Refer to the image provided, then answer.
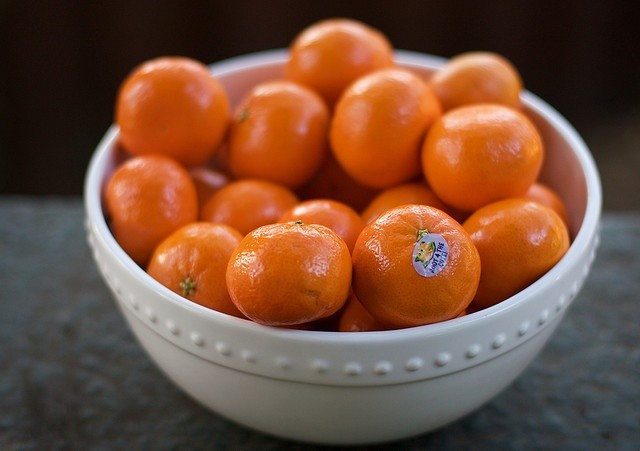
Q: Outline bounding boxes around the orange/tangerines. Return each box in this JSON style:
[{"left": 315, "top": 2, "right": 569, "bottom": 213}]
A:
[{"left": 103, "top": 16, "right": 571, "bottom": 332}]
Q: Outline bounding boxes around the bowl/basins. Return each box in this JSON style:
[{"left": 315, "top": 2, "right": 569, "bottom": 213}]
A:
[{"left": 82, "top": 52, "right": 602, "bottom": 443}]
